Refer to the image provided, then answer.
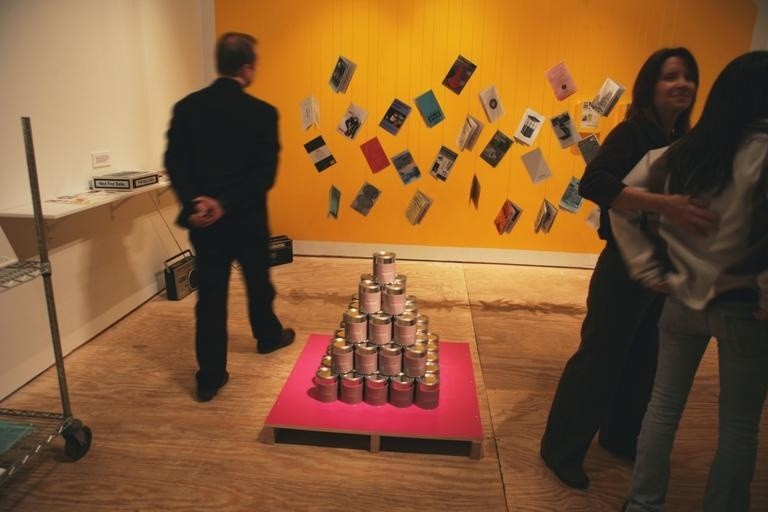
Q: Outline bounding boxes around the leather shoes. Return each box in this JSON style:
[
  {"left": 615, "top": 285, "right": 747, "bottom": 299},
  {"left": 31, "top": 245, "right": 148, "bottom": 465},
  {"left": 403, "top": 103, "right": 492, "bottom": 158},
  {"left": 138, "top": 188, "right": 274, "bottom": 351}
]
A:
[
  {"left": 541, "top": 433, "right": 636, "bottom": 488},
  {"left": 258, "top": 329, "right": 294, "bottom": 353},
  {"left": 197, "top": 371, "right": 228, "bottom": 401}
]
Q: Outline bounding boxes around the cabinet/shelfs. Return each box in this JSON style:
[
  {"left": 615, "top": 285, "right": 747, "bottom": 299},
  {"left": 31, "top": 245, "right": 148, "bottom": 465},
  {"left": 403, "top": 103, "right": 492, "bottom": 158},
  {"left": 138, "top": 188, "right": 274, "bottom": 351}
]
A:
[{"left": 0, "top": 116, "right": 94, "bottom": 490}]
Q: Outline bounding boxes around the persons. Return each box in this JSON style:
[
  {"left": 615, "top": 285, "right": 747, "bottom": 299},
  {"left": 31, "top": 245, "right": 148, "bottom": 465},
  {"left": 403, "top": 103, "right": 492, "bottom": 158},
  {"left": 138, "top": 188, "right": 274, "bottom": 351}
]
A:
[
  {"left": 609, "top": 50, "right": 768, "bottom": 512},
  {"left": 163, "top": 33, "right": 295, "bottom": 402},
  {"left": 540, "top": 47, "right": 720, "bottom": 488}
]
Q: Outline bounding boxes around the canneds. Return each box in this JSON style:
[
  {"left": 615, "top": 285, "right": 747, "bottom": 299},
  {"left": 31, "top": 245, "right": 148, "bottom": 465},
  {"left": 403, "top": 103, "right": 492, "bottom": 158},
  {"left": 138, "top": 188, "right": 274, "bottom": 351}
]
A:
[{"left": 316, "top": 251, "right": 438, "bottom": 409}]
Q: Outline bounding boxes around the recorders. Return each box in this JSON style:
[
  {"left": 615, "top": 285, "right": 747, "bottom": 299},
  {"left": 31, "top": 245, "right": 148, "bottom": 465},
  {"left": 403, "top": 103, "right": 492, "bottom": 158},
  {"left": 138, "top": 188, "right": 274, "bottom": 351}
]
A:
[
  {"left": 163, "top": 249, "right": 201, "bottom": 301},
  {"left": 268, "top": 234, "right": 293, "bottom": 267}
]
